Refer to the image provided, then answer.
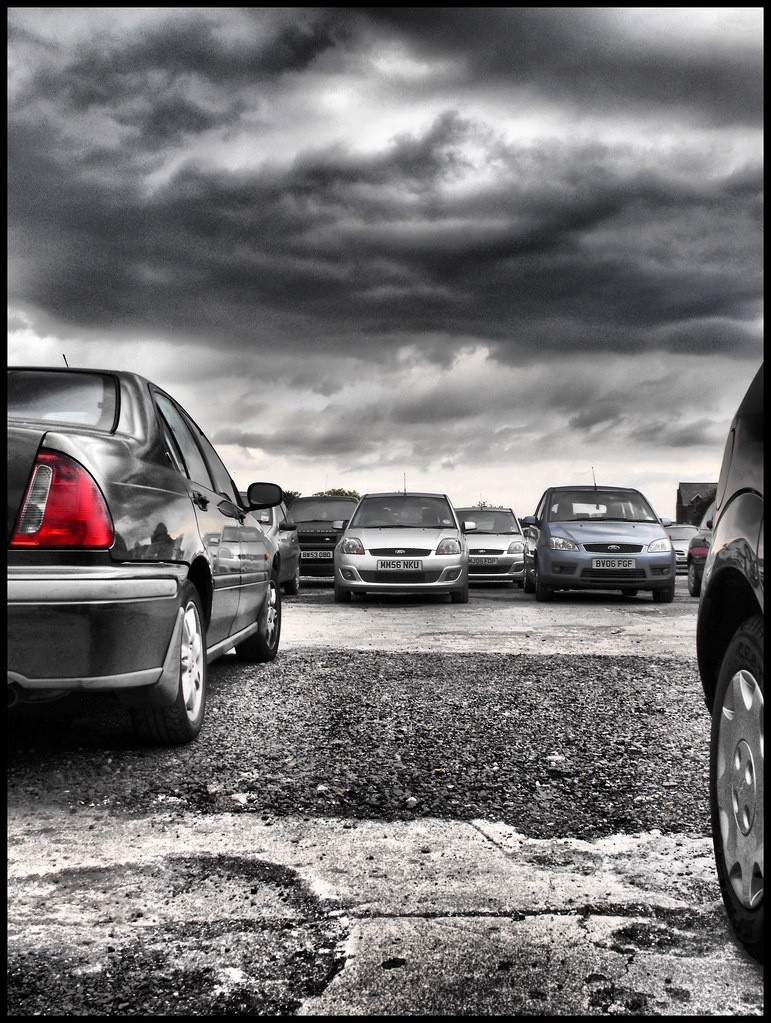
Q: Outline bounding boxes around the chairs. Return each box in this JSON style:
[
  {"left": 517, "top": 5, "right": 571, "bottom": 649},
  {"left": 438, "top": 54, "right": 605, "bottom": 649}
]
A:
[
  {"left": 607, "top": 504, "right": 629, "bottom": 519},
  {"left": 365, "top": 510, "right": 387, "bottom": 521},
  {"left": 557, "top": 500, "right": 576, "bottom": 520},
  {"left": 492, "top": 516, "right": 510, "bottom": 531},
  {"left": 423, "top": 507, "right": 439, "bottom": 522}
]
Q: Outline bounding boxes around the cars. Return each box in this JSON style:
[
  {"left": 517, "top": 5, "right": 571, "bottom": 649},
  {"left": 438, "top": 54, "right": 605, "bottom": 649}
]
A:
[
  {"left": 452, "top": 505, "right": 529, "bottom": 590},
  {"left": 331, "top": 491, "right": 478, "bottom": 604},
  {"left": 663, "top": 523, "right": 699, "bottom": 569},
  {"left": 695, "top": 357, "right": 766, "bottom": 964},
  {"left": 687, "top": 500, "right": 717, "bottom": 597},
  {"left": 279, "top": 494, "right": 362, "bottom": 576},
  {"left": 523, "top": 485, "right": 677, "bottom": 603},
  {"left": 237, "top": 490, "right": 301, "bottom": 595},
  {"left": 6, "top": 365, "right": 283, "bottom": 750}
]
